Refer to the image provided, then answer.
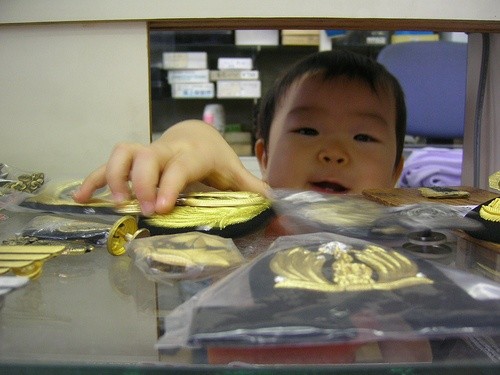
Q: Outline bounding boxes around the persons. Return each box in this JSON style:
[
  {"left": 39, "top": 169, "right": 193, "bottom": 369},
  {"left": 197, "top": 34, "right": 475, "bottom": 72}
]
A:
[{"left": 72, "top": 50, "right": 407, "bottom": 220}]
